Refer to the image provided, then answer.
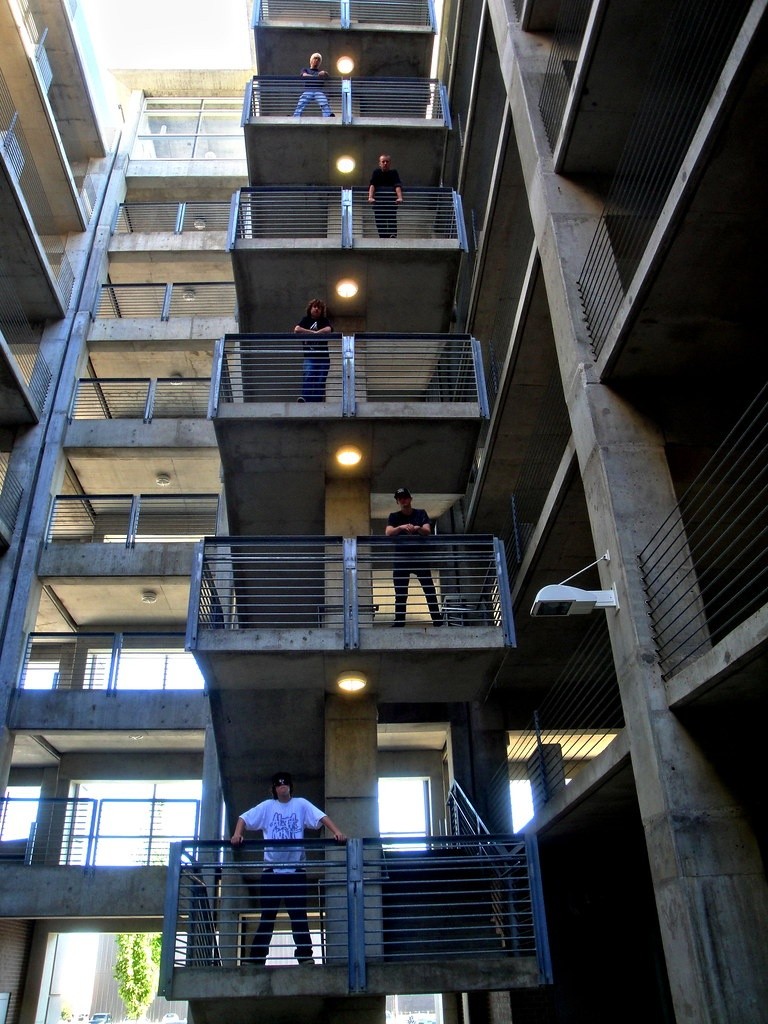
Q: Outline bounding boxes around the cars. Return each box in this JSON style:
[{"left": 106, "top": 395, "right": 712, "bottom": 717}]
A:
[
  {"left": 162, "top": 1013, "right": 181, "bottom": 1024},
  {"left": 88, "top": 1011, "right": 115, "bottom": 1024}
]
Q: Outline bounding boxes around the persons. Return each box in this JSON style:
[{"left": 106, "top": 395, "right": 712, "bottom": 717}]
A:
[
  {"left": 293, "top": 53, "right": 336, "bottom": 117},
  {"left": 368, "top": 151, "right": 402, "bottom": 238},
  {"left": 231, "top": 772, "right": 347, "bottom": 965},
  {"left": 294, "top": 298, "right": 333, "bottom": 402},
  {"left": 385, "top": 487, "right": 444, "bottom": 627}
]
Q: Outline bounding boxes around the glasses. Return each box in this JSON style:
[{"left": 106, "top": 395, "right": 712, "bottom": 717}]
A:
[{"left": 275, "top": 780, "right": 291, "bottom": 786}]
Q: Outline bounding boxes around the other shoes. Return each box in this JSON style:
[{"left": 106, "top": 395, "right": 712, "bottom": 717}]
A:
[
  {"left": 298, "top": 956, "right": 314, "bottom": 965},
  {"left": 241, "top": 961, "right": 264, "bottom": 966},
  {"left": 391, "top": 624, "right": 404, "bottom": 627},
  {"left": 297, "top": 398, "right": 305, "bottom": 402},
  {"left": 389, "top": 234, "right": 396, "bottom": 238}
]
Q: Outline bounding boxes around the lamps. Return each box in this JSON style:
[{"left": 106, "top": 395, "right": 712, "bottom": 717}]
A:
[
  {"left": 194, "top": 219, "right": 207, "bottom": 230},
  {"left": 169, "top": 375, "right": 183, "bottom": 386},
  {"left": 204, "top": 151, "right": 216, "bottom": 159},
  {"left": 182, "top": 290, "right": 194, "bottom": 302},
  {"left": 141, "top": 592, "right": 157, "bottom": 604},
  {"left": 530, "top": 550, "right": 621, "bottom": 618},
  {"left": 155, "top": 474, "right": 171, "bottom": 486},
  {"left": 336, "top": 671, "right": 368, "bottom": 692}
]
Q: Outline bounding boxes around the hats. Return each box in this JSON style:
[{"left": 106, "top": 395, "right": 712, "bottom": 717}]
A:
[{"left": 394, "top": 487, "right": 412, "bottom": 498}]
